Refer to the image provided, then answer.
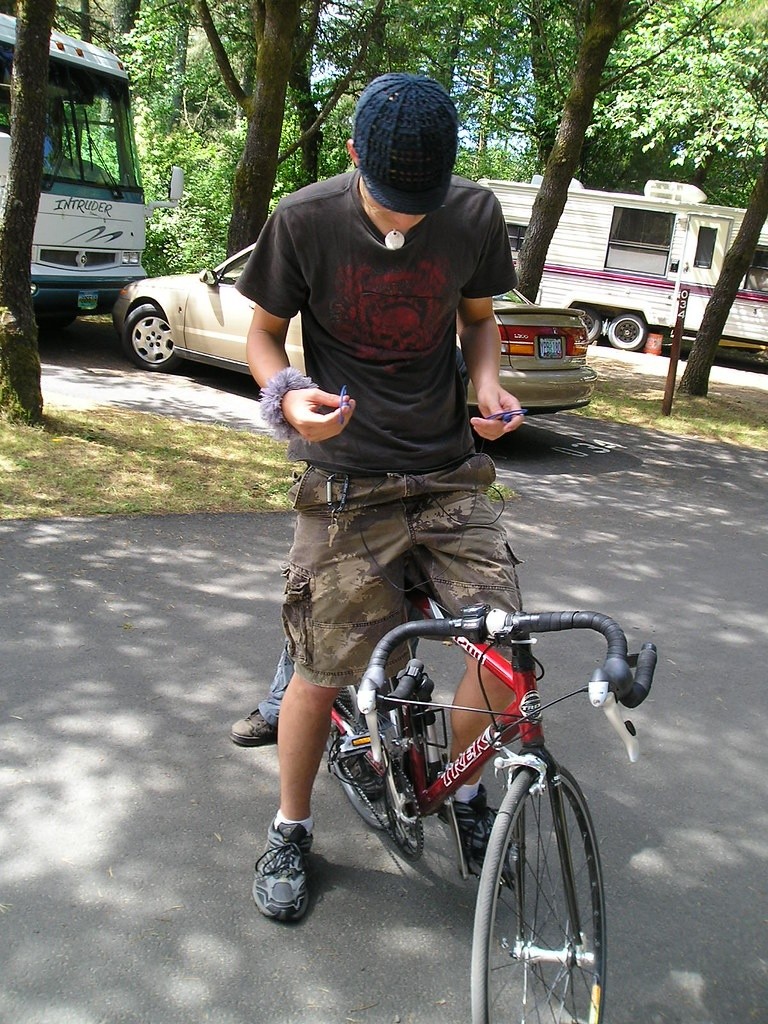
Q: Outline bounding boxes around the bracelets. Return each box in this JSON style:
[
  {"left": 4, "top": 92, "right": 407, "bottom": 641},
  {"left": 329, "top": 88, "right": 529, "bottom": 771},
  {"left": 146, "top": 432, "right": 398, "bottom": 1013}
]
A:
[{"left": 258, "top": 364, "right": 319, "bottom": 441}]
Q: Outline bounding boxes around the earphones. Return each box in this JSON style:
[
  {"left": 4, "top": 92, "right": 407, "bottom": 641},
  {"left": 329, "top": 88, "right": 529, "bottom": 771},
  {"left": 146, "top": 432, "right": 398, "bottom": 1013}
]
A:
[
  {"left": 341, "top": 388, "right": 350, "bottom": 406},
  {"left": 502, "top": 413, "right": 513, "bottom": 423}
]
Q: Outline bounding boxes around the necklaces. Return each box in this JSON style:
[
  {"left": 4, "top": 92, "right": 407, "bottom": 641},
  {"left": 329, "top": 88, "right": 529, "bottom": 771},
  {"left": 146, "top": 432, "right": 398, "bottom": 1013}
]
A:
[{"left": 364, "top": 195, "right": 404, "bottom": 250}]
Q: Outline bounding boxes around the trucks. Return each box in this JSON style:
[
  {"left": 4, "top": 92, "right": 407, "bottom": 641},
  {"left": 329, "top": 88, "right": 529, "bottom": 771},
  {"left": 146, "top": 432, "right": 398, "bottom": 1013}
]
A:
[
  {"left": 0, "top": 13, "right": 180, "bottom": 346},
  {"left": 474, "top": 173, "right": 768, "bottom": 370}
]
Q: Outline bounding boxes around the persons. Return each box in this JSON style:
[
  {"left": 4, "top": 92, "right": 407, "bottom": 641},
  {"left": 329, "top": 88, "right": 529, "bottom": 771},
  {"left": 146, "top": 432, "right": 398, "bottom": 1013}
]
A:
[{"left": 229, "top": 72, "right": 524, "bottom": 924}]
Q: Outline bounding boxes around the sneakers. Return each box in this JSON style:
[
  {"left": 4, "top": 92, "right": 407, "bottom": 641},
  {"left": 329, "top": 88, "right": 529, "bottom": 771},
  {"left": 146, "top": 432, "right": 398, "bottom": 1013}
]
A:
[
  {"left": 253, "top": 814, "right": 313, "bottom": 921},
  {"left": 229, "top": 708, "right": 279, "bottom": 747},
  {"left": 439, "top": 784, "right": 519, "bottom": 874}
]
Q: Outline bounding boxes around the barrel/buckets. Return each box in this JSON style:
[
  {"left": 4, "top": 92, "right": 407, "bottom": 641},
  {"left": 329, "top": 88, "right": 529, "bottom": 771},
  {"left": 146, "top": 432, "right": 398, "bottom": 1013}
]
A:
[{"left": 643, "top": 333, "right": 664, "bottom": 354}]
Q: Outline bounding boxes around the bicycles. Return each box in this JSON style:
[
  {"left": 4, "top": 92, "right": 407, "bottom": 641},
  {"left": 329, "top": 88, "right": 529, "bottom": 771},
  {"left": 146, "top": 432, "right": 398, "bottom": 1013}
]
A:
[{"left": 327, "top": 544, "right": 661, "bottom": 1024}]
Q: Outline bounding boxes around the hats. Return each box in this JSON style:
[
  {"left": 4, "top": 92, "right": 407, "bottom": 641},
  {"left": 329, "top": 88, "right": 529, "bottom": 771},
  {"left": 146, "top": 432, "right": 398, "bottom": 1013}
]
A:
[{"left": 353, "top": 72, "right": 457, "bottom": 214}]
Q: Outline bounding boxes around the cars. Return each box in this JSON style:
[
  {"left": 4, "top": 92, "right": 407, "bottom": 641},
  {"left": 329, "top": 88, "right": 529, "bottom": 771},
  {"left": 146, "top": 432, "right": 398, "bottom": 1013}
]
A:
[{"left": 114, "top": 240, "right": 597, "bottom": 443}]
